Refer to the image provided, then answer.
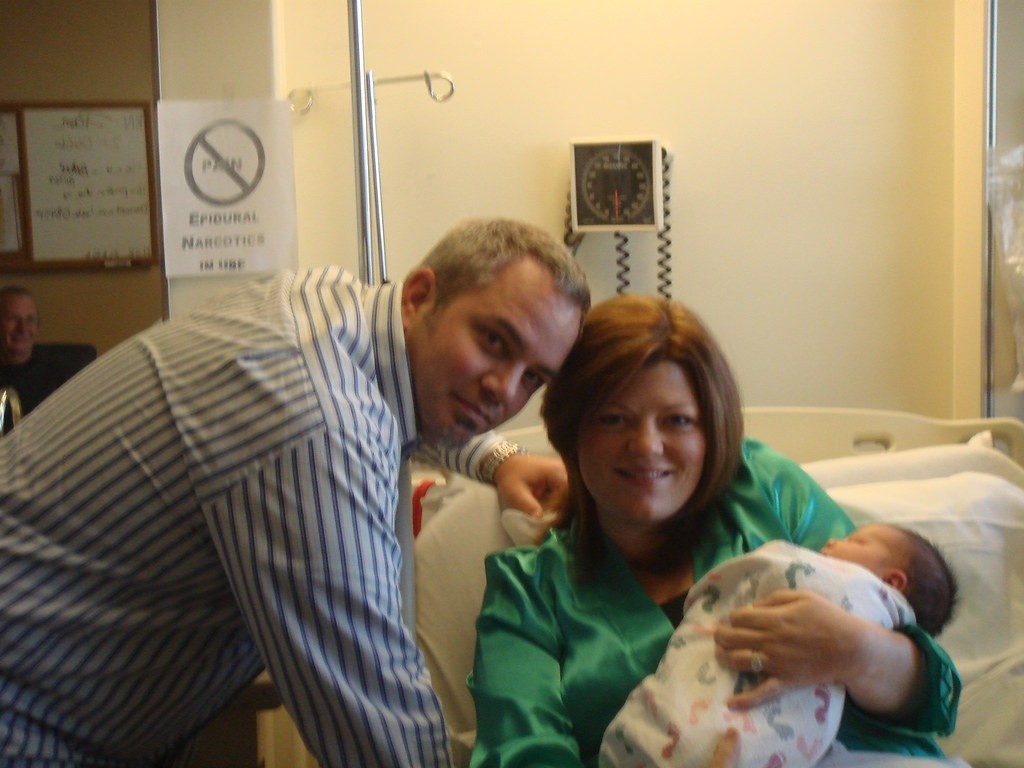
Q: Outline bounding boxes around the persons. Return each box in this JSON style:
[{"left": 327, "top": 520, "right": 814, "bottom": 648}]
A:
[
  {"left": 465, "top": 290, "right": 963, "bottom": 768},
  {"left": 0, "top": 220, "right": 591, "bottom": 768},
  {"left": 0, "top": 284, "right": 43, "bottom": 437}
]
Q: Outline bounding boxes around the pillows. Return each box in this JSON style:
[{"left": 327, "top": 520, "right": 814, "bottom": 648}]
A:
[{"left": 415, "top": 432, "right": 1024, "bottom": 768}]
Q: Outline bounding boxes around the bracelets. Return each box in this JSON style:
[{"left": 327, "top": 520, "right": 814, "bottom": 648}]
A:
[{"left": 483, "top": 441, "right": 521, "bottom": 484}]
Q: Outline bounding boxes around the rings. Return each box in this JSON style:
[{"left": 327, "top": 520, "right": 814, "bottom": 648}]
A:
[{"left": 751, "top": 648, "right": 763, "bottom": 673}]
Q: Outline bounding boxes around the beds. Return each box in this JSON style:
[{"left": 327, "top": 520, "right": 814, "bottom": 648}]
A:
[{"left": 410, "top": 406, "right": 1024, "bottom": 768}]
